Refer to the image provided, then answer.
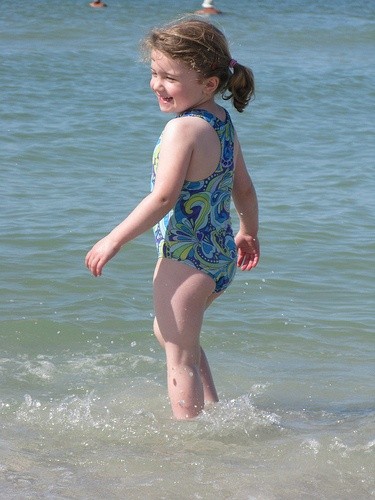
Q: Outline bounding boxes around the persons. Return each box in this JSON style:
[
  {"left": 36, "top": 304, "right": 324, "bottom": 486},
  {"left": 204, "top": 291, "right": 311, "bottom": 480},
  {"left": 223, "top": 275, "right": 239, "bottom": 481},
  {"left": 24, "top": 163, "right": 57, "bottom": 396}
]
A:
[
  {"left": 195, "top": 0, "right": 223, "bottom": 14},
  {"left": 89, "top": 0, "right": 107, "bottom": 8},
  {"left": 84, "top": 19, "right": 260, "bottom": 421}
]
